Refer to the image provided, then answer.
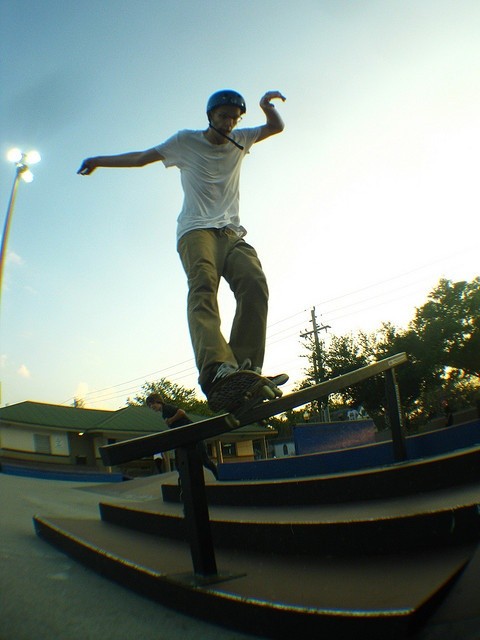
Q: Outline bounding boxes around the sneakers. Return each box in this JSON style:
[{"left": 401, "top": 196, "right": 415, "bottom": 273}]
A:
[
  {"left": 223, "top": 373, "right": 289, "bottom": 414},
  {"left": 200, "top": 359, "right": 261, "bottom": 394}
]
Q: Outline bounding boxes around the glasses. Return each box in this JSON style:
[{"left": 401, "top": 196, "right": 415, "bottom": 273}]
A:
[{"left": 214, "top": 111, "right": 242, "bottom": 123}]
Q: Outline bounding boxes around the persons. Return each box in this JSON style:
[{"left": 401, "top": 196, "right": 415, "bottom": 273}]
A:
[
  {"left": 144, "top": 392, "right": 219, "bottom": 483},
  {"left": 442, "top": 401, "right": 454, "bottom": 427},
  {"left": 75, "top": 89, "right": 290, "bottom": 408},
  {"left": 152, "top": 452, "right": 163, "bottom": 476}
]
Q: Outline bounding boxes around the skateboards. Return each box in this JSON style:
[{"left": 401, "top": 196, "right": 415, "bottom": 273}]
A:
[{"left": 207, "top": 370, "right": 283, "bottom": 429}]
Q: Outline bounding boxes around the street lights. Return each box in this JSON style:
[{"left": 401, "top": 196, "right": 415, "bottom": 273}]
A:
[{"left": 0, "top": 149, "right": 41, "bottom": 286}]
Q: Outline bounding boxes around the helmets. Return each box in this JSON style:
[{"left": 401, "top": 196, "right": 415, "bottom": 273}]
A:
[{"left": 207, "top": 89, "right": 246, "bottom": 115}]
